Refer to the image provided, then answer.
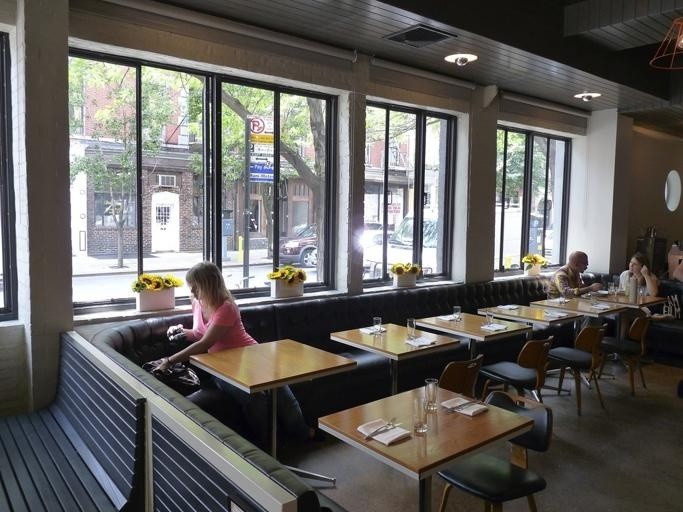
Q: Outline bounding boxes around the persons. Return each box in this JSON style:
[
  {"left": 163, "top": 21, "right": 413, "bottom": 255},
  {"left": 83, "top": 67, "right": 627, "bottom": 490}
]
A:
[
  {"left": 617, "top": 252, "right": 658, "bottom": 341},
  {"left": 153, "top": 264, "right": 325, "bottom": 447},
  {"left": 546, "top": 252, "right": 602, "bottom": 338}
]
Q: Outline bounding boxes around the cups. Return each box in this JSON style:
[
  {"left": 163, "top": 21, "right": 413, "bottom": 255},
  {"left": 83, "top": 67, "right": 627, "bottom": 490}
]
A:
[
  {"left": 485, "top": 308, "right": 493, "bottom": 329},
  {"left": 591, "top": 292, "right": 597, "bottom": 305},
  {"left": 453, "top": 306, "right": 461, "bottom": 324},
  {"left": 424, "top": 379, "right": 438, "bottom": 412},
  {"left": 559, "top": 291, "right": 565, "bottom": 305},
  {"left": 638, "top": 287, "right": 644, "bottom": 300},
  {"left": 412, "top": 397, "right": 429, "bottom": 433},
  {"left": 373, "top": 317, "right": 382, "bottom": 337},
  {"left": 608, "top": 282, "right": 614, "bottom": 295},
  {"left": 407, "top": 318, "right": 416, "bottom": 341}
]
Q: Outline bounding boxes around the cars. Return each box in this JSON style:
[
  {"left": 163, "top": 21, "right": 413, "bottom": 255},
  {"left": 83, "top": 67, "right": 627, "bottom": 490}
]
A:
[{"left": 268, "top": 222, "right": 383, "bottom": 267}]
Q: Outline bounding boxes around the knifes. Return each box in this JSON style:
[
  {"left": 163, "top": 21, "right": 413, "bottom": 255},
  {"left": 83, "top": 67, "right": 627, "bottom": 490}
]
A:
[{"left": 367, "top": 422, "right": 403, "bottom": 440}]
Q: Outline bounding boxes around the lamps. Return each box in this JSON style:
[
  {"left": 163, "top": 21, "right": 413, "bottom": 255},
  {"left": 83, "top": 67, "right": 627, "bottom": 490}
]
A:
[
  {"left": 648, "top": 14, "right": 683, "bottom": 74},
  {"left": 443, "top": 52, "right": 479, "bottom": 68},
  {"left": 572, "top": 92, "right": 602, "bottom": 103}
]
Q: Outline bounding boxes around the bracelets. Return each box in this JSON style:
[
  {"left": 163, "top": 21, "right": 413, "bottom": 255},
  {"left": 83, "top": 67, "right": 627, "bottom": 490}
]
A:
[{"left": 166, "top": 358, "right": 173, "bottom": 366}]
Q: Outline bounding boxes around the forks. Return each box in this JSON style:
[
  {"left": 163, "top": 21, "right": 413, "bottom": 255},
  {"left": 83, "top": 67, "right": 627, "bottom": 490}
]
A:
[
  {"left": 447, "top": 400, "right": 487, "bottom": 414},
  {"left": 364, "top": 418, "right": 400, "bottom": 444}
]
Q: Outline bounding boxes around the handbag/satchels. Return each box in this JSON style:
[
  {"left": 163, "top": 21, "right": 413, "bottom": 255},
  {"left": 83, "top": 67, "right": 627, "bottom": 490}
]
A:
[{"left": 146, "top": 360, "right": 201, "bottom": 395}]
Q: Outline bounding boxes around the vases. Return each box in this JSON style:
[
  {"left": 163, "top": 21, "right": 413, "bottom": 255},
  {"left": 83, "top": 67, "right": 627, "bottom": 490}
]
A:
[
  {"left": 523, "top": 263, "right": 542, "bottom": 276},
  {"left": 135, "top": 287, "right": 177, "bottom": 314},
  {"left": 270, "top": 279, "right": 304, "bottom": 299},
  {"left": 392, "top": 274, "right": 417, "bottom": 288}
]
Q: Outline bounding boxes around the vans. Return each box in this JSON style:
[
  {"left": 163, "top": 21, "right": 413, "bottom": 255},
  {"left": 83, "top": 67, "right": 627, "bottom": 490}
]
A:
[{"left": 365, "top": 206, "right": 521, "bottom": 280}]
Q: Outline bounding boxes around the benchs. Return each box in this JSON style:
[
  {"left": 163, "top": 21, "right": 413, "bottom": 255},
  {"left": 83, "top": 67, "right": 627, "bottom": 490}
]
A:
[{"left": 0, "top": 330, "right": 300, "bottom": 512}]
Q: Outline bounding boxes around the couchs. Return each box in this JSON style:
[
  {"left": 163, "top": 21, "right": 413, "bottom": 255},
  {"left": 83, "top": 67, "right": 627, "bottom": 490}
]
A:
[{"left": 75, "top": 268, "right": 682, "bottom": 512}]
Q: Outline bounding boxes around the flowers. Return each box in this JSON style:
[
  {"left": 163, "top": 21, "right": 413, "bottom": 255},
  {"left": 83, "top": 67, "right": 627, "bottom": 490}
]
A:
[
  {"left": 132, "top": 273, "right": 183, "bottom": 293},
  {"left": 390, "top": 262, "right": 422, "bottom": 276},
  {"left": 266, "top": 265, "right": 307, "bottom": 286},
  {"left": 521, "top": 253, "right": 551, "bottom": 269}
]
